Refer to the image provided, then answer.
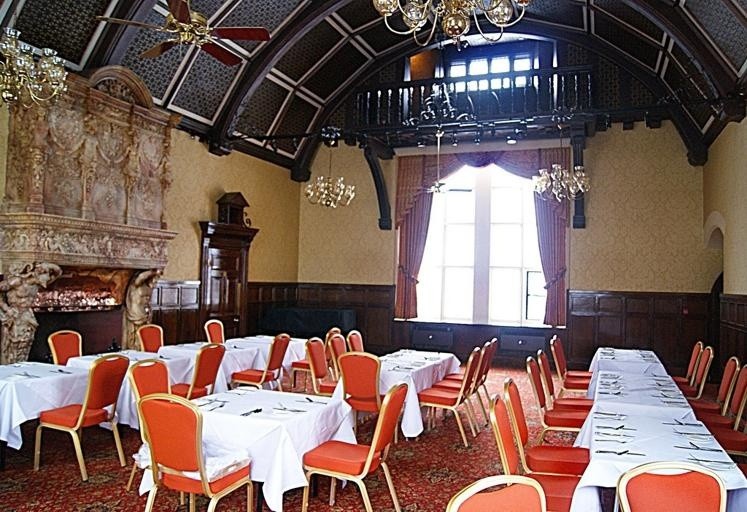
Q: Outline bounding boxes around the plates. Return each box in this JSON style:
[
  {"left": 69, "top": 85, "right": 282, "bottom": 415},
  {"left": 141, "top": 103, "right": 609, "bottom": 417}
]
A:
[
  {"left": 377, "top": 349, "right": 441, "bottom": 372},
  {"left": 95, "top": 349, "right": 160, "bottom": 364}
]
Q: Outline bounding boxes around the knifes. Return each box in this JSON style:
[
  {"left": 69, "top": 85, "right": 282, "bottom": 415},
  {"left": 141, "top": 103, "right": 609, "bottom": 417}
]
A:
[
  {"left": 662, "top": 419, "right": 738, "bottom": 470},
  {"left": 14, "top": 362, "right": 72, "bottom": 378},
  {"left": 594, "top": 411, "right": 646, "bottom": 472}
]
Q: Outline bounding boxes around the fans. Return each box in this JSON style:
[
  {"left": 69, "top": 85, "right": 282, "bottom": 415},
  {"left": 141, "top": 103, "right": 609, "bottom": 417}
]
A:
[
  {"left": 396, "top": 129, "right": 474, "bottom": 199},
  {"left": 89, "top": 0, "right": 271, "bottom": 68}
]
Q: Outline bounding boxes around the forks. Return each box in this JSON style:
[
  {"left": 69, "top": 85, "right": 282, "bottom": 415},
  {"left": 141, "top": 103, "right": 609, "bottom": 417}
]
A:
[
  {"left": 234, "top": 346, "right": 243, "bottom": 349},
  {"left": 158, "top": 355, "right": 171, "bottom": 360},
  {"left": 199, "top": 387, "right": 327, "bottom": 417},
  {"left": 598, "top": 347, "right": 688, "bottom": 408}
]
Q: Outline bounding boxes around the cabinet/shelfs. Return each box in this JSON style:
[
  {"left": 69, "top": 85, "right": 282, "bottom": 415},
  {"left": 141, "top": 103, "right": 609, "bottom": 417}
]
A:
[{"left": 391, "top": 317, "right": 568, "bottom": 370}]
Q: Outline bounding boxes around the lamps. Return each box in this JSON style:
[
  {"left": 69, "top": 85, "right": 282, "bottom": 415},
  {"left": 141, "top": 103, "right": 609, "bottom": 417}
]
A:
[
  {"left": 524, "top": 105, "right": 591, "bottom": 204},
  {"left": 372, "top": 0, "right": 532, "bottom": 52},
  {"left": 0, "top": 9, "right": 70, "bottom": 113},
  {"left": 303, "top": 126, "right": 357, "bottom": 209}
]
